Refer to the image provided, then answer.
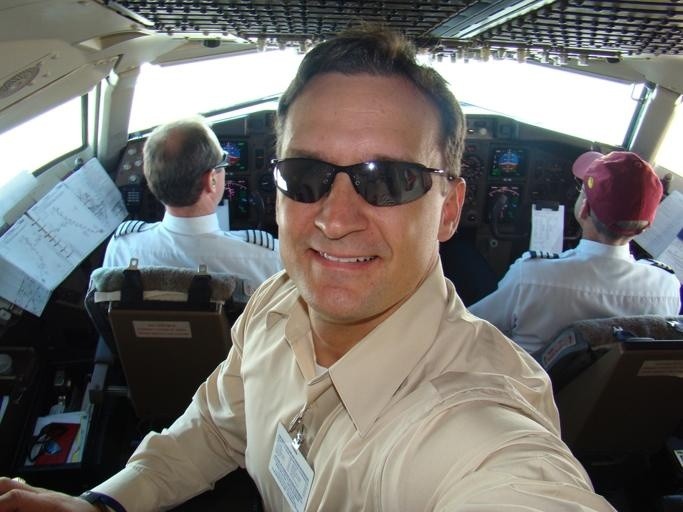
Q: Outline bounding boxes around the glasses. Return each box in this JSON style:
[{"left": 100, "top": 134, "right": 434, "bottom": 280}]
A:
[
  {"left": 269, "top": 155, "right": 455, "bottom": 208},
  {"left": 202, "top": 149, "right": 231, "bottom": 177}
]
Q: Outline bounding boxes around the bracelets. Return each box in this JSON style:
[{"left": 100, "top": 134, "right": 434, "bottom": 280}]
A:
[{"left": 79, "top": 490, "right": 126, "bottom": 512}]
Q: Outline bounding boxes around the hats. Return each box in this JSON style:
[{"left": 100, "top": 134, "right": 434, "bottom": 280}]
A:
[{"left": 573, "top": 149, "right": 665, "bottom": 236}]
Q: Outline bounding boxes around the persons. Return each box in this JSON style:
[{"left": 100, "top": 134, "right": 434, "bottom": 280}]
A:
[
  {"left": 465, "top": 148, "right": 682, "bottom": 356},
  {"left": 1, "top": 25, "right": 623, "bottom": 512},
  {"left": 100, "top": 112, "right": 282, "bottom": 304}
]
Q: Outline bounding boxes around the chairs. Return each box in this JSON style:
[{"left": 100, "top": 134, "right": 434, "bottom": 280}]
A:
[
  {"left": 531, "top": 316, "right": 683, "bottom": 458},
  {"left": 84, "top": 264, "right": 256, "bottom": 421}
]
